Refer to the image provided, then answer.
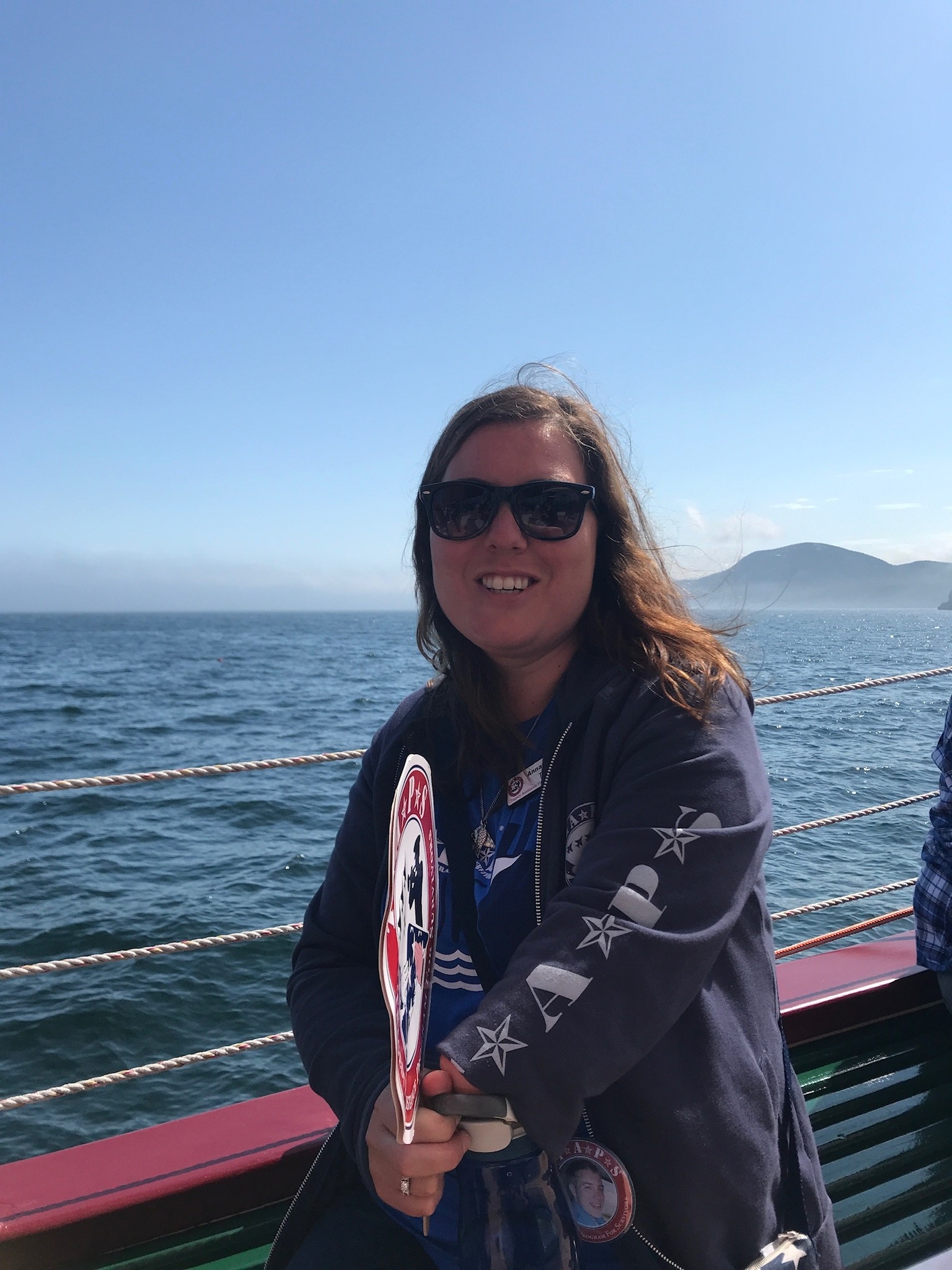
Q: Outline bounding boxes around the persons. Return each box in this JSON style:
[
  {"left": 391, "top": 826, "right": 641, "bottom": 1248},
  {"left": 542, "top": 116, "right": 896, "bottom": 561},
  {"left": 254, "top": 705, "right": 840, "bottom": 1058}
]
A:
[
  {"left": 912, "top": 695, "right": 952, "bottom": 1016},
  {"left": 565, "top": 1159, "right": 607, "bottom": 1226},
  {"left": 260, "top": 382, "right": 843, "bottom": 1270}
]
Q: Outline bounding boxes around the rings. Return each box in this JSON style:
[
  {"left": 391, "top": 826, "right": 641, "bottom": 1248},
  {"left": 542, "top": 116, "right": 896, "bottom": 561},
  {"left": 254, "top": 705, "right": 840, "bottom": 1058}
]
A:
[{"left": 400, "top": 1175, "right": 411, "bottom": 1196}]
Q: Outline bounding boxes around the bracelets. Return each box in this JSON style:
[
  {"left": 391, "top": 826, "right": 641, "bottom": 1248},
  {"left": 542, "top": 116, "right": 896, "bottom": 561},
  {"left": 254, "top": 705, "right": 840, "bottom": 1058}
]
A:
[{"left": 424, "top": 1092, "right": 516, "bottom": 1125}]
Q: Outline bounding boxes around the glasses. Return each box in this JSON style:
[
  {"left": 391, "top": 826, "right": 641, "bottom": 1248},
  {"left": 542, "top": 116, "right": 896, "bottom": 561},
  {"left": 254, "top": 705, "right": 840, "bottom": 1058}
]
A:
[{"left": 419, "top": 479, "right": 601, "bottom": 540}]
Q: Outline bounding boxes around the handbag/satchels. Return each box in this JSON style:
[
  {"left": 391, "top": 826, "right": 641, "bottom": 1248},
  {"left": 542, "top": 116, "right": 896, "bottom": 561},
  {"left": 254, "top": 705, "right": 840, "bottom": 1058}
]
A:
[{"left": 743, "top": 1229, "right": 820, "bottom": 1270}]
